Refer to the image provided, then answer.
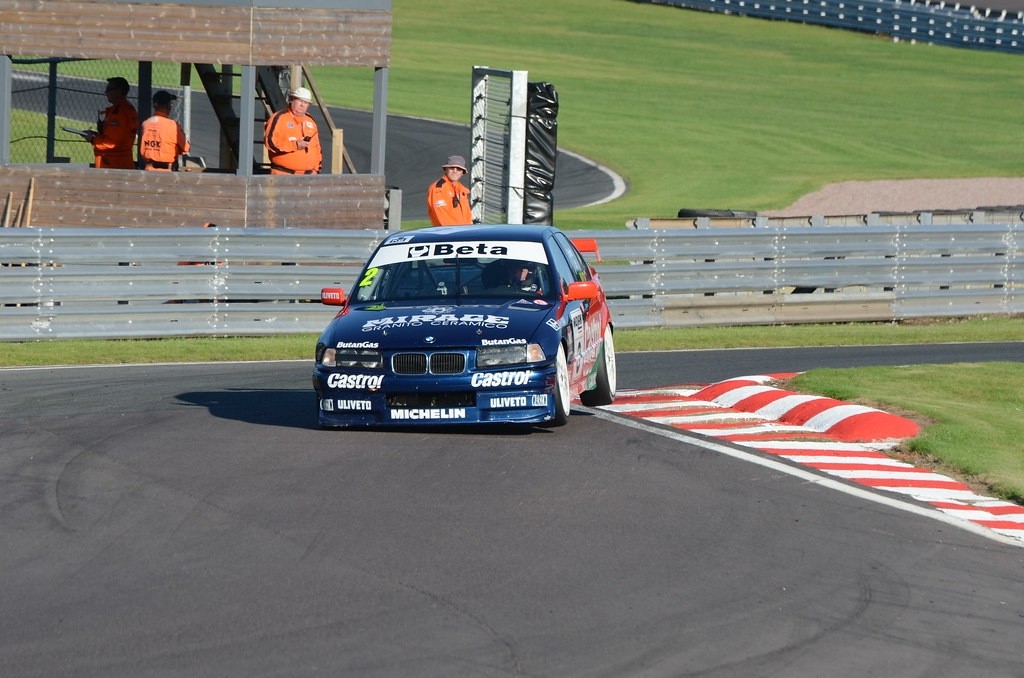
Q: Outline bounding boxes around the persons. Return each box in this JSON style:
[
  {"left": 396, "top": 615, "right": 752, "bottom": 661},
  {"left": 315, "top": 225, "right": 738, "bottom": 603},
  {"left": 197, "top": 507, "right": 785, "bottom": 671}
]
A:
[
  {"left": 140, "top": 90, "right": 191, "bottom": 171},
  {"left": 265, "top": 87, "right": 323, "bottom": 176},
  {"left": 80, "top": 77, "right": 138, "bottom": 169},
  {"left": 427, "top": 155, "right": 472, "bottom": 227},
  {"left": 502, "top": 260, "right": 543, "bottom": 295}
]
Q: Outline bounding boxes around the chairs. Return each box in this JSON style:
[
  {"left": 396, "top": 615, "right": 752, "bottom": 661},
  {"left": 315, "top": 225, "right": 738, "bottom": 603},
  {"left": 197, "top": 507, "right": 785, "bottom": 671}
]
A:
[{"left": 571, "top": 238, "right": 601, "bottom": 263}]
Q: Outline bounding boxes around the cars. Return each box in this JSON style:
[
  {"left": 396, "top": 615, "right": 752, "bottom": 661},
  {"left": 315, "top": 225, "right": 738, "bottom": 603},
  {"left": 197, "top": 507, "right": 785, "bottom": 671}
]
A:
[{"left": 312, "top": 224, "right": 620, "bottom": 436}]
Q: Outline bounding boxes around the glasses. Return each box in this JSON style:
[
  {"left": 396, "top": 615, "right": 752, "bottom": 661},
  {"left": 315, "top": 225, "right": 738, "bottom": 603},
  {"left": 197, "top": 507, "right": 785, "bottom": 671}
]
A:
[{"left": 449, "top": 167, "right": 462, "bottom": 172}]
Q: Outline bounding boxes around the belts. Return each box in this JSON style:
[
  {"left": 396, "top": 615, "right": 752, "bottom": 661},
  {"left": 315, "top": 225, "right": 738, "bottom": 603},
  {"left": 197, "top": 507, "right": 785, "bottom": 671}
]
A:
[
  {"left": 271, "top": 163, "right": 313, "bottom": 175},
  {"left": 145, "top": 162, "right": 172, "bottom": 169}
]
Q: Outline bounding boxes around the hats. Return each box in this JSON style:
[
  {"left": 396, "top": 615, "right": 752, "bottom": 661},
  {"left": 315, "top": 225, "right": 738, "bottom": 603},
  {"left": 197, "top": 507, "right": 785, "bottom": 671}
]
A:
[
  {"left": 290, "top": 87, "right": 315, "bottom": 104},
  {"left": 153, "top": 90, "right": 177, "bottom": 102},
  {"left": 442, "top": 155, "right": 468, "bottom": 174}
]
